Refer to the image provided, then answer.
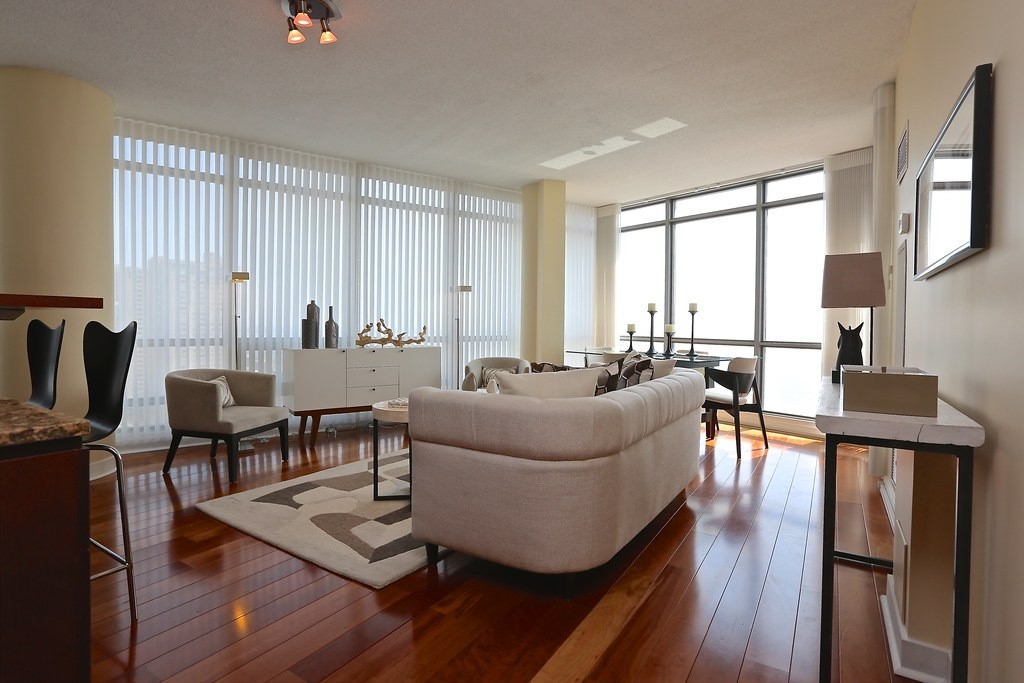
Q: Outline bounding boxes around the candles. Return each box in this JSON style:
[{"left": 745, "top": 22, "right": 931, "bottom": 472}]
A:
[{"left": 628, "top": 303, "right": 698, "bottom": 332}]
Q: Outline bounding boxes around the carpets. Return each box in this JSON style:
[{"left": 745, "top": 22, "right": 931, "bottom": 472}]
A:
[{"left": 195, "top": 447, "right": 454, "bottom": 590}]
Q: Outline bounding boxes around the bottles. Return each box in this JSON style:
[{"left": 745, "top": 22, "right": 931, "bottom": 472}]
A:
[{"left": 302, "top": 299, "right": 339, "bottom": 349}]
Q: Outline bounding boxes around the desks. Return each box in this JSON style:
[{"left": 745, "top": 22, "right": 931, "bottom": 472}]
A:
[
  {"left": 567, "top": 350, "right": 763, "bottom": 438},
  {"left": 816, "top": 375, "right": 986, "bottom": 683}
]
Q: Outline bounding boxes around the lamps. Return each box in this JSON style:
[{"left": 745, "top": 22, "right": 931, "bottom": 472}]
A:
[
  {"left": 456, "top": 285, "right": 472, "bottom": 389},
  {"left": 227, "top": 271, "right": 253, "bottom": 451},
  {"left": 280, "top": 0, "right": 341, "bottom": 45},
  {"left": 821, "top": 251, "right": 886, "bottom": 366}
]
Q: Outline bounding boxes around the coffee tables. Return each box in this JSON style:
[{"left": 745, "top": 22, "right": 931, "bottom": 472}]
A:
[{"left": 372, "top": 399, "right": 411, "bottom": 511}]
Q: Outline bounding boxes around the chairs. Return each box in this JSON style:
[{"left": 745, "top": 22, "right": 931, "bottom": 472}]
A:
[
  {"left": 163, "top": 368, "right": 289, "bottom": 482},
  {"left": 703, "top": 355, "right": 769, "bottom": 458},
  {"left": 584, "top": 346, "right": 615, "bottom": 368}
]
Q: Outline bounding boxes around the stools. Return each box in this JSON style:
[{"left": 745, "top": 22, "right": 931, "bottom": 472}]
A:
[
  {"left": 82, "top": 321, "right": 138, "bottom": 620},
  {"left": 27, "top": 318, "right": 66, "bottom": 410}
]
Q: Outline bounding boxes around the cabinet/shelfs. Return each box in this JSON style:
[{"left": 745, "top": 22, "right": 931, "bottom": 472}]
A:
[{"left": 282, "top": 347, "right": 442, "bottom": 446}]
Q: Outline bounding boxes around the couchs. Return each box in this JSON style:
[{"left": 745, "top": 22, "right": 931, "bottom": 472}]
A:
[
  {"left": 408, "top": 362, "right": 708, "bottom": 594},
  {"left": 464, "top": 357, "right": 532, "bottom": 389}
]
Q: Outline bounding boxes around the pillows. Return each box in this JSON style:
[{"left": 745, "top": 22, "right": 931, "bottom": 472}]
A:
[
  {"left": 482, "top": 350, "right": 677, "bottom": 399},
  {"left": 209, "top": 376, "right": 237, "bottom": 408}
]
